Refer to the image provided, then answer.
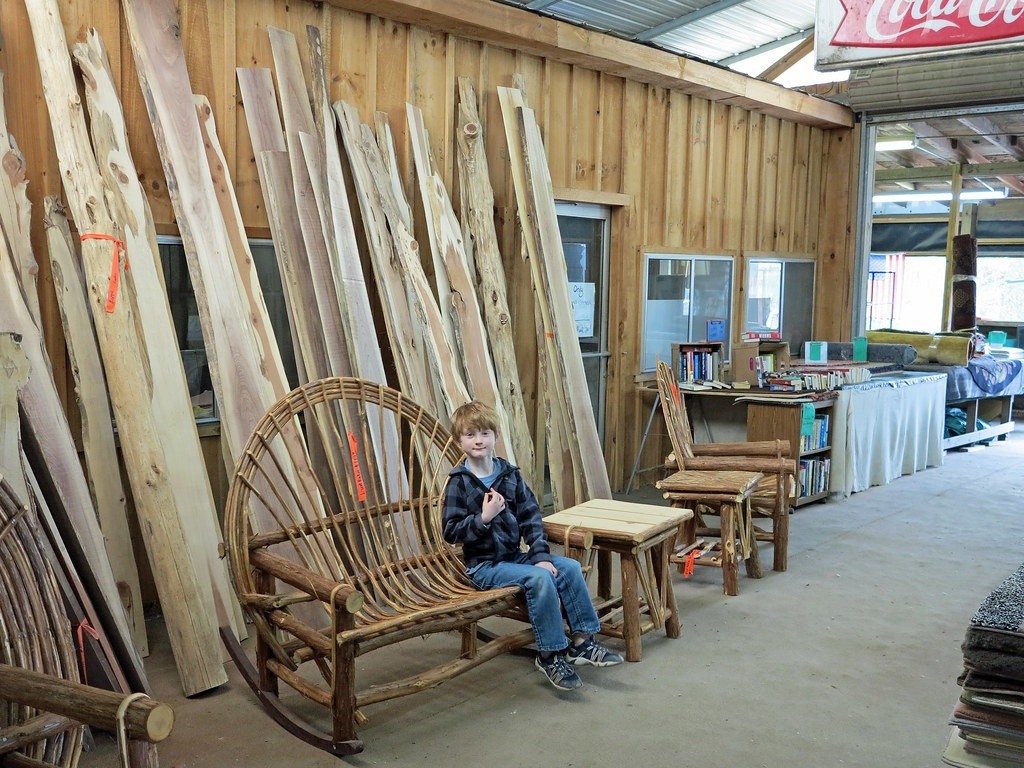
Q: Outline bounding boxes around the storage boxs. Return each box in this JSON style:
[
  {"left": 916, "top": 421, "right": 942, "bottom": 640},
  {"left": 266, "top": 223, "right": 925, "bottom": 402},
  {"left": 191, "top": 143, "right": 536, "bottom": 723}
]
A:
[
  {"left": 671, "top": 343, "right": 725, "bottom": 383},
  {"left": 731, "top": 339, "right": 791, "bottom": 385}
]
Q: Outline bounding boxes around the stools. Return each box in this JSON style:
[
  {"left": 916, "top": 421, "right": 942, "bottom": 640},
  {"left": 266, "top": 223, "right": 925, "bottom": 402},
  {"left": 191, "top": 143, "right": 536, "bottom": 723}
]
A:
[
  {"left": 540, "top": 497, "right": 694, "bottom": 663},
  {"left": 656, "top": 471, "right": 764, "bottom": 597}
]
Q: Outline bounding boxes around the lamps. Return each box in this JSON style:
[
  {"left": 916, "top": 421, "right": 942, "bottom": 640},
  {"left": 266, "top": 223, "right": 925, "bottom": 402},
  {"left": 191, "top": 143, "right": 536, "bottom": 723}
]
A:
[
  {"left": 871, "top": 187, "right": 1009, "bottom": 203},
  {"left": 875, "top": 134, "right": 919, "bottom": 152}
]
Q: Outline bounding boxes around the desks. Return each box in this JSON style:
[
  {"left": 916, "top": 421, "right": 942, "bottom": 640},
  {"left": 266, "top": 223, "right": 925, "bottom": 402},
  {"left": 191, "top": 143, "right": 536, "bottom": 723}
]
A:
[
  {"left": 825, "top": 370, "right": 949, "bottom": 492},
  {"left": 904, "top": 359, "right": 1024, "bottom": 457},
  {"left": 625, "top": 384, "right": 834, "bottom": 515}
]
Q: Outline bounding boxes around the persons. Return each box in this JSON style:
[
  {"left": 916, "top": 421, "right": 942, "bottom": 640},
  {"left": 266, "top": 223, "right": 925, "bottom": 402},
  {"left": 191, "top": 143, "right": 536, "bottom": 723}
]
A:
[{"left": 441, "top": 401, "right": 624, "bottom": 690}]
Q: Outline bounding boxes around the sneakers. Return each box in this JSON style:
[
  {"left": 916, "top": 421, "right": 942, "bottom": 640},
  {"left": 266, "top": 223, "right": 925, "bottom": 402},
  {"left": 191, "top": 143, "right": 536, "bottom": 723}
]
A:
[
  {"left": 535, "top": 651, "right": 583, "bottom": 691},
  {"left": 565, "top": 635, "right": 624, "bottom": 667}
]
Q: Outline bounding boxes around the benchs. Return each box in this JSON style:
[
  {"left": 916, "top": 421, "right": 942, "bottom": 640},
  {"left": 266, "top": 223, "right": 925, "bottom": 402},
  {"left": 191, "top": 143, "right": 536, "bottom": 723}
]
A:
[
  {"left": 1, "top": 485, "right": 178, "bottom": 768},
  {"left": 216, "top": 375, "right": 592, "bottom": 757}
]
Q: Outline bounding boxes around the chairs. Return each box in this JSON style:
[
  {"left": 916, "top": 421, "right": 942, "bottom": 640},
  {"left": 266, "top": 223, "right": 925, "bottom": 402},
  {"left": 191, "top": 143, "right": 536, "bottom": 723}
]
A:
[{"left": 651, "top": 360, "right": 796, "bottom": 573}]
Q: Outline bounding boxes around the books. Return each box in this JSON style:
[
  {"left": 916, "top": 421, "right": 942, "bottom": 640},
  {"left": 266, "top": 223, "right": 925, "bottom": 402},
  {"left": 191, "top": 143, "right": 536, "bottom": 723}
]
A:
[
  {"left": 799, "top": 458, "right": 830, "bottom": 496},
  {"left": 800, "top": 414, "right": 829, "bottom": 452},
  {"left": 678, "top": 330, "right": 874, "bottom": 392}
]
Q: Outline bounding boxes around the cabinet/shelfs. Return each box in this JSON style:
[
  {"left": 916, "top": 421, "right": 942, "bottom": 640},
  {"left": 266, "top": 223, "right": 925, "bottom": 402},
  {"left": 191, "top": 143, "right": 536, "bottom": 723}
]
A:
[{"left": 748, "top": 401, "right": 833, "bottom": 515}]
